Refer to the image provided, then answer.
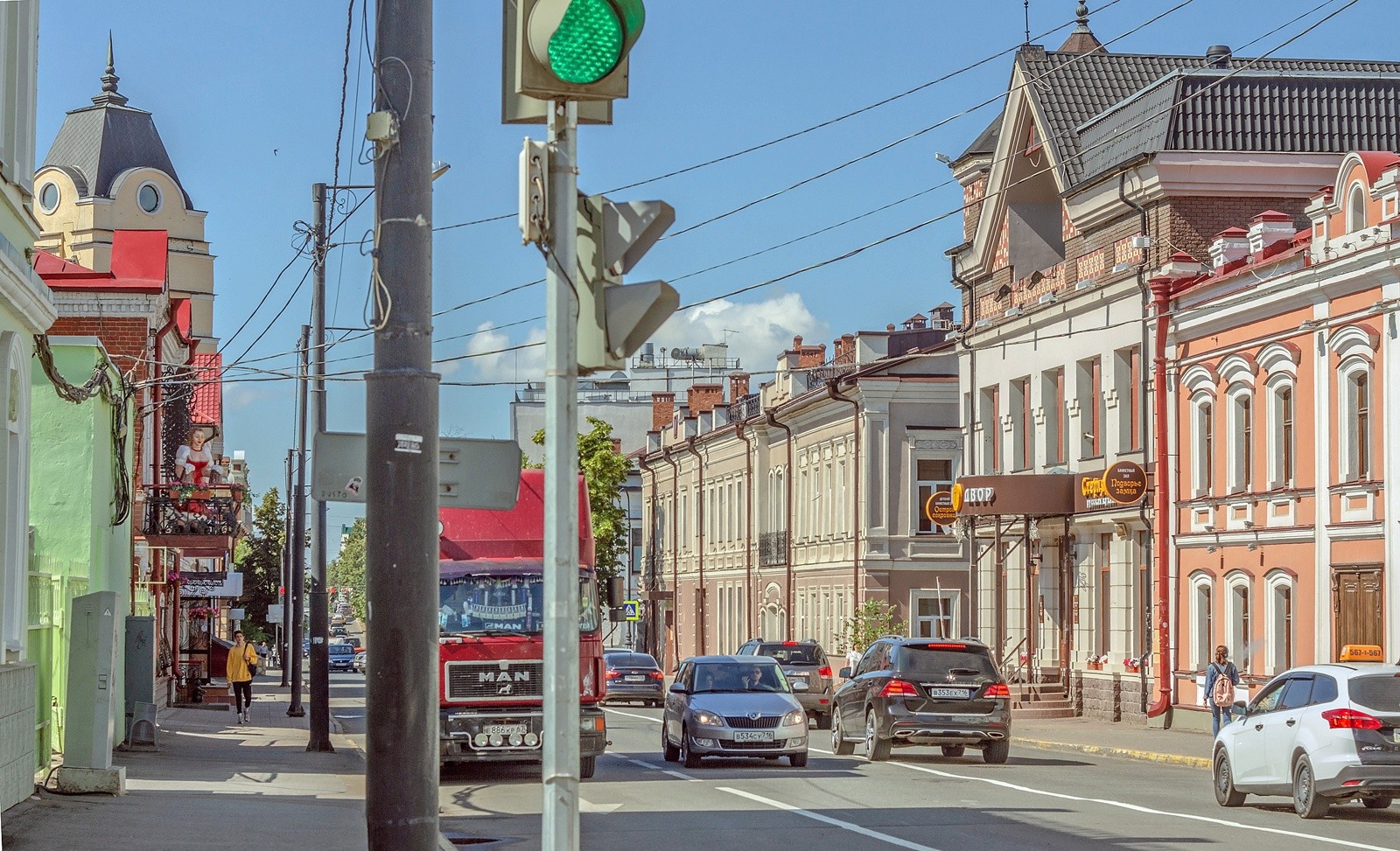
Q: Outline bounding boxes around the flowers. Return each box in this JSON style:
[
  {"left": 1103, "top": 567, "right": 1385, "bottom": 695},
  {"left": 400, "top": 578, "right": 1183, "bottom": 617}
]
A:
[
  {"left": 189, "top": 607, "right": 219, "bottom": 620},
  {"left": 1085, "top": 654, "right": 1107, "bottom": 664},
  {"left": 169, "top": 481, "right": 210, "bottom": 506},
  {"left": 1124, "top": 657, "right": 1149, "bottom": 668},
  {"left": 231, "top": 483, "right": 246, "bottom": 494},
  {"left": 1020, "top": 651, "right": 1036, "bottom": 661},
  {"left": 168, "top": 573, "right": 188, "bottom": 584}
]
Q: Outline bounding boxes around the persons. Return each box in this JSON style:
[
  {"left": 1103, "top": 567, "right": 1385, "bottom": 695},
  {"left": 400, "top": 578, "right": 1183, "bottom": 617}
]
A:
[
  {"left": 226, "top": 630, "right": 257, "bottom": 724},
  {"left": 844, "top": 641, "right": 864, "bottom": 678},
  {"left": 1203, "top": 645, "right": 1240, "bottom": 740},
  {"left": 741, "top": 666, "right": 776, "bottom": 693},
  {"left": 271, "top": 644, "right": 276, "bottom": 667},
  {"left": 215, "top": 456, "right": 235, "bottom": 486},
  {"left": 259, "top": 641, "right": 268, "bottom": 658},
  {"left": 175, "top": 427, "right": 213, "bottom": 533},
  {"left": 702, "top": 671, "right": 715, "bottom": 689}
]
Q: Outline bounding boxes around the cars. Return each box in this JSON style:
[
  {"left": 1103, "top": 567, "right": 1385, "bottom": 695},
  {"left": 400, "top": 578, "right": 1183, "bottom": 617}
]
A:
[
  {"left": 599, "top": 647, "right": 666, "bottom": 707},
  {"left": 303, "top": 612, "right": 367, "bottom": 674},
  {"left": 661, "top": 655, "right": 809, "bottom": 768},
  {"left": 1211, "top": 645, "right": 1400, "bottom": 818}
]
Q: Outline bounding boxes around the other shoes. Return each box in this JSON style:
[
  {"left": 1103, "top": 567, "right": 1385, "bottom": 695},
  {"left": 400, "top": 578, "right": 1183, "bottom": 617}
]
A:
[
  {"left": 238, "top": 716, "right": 244, "bottom": 722},
  {"left": 243, "top": 709, "right": 250, "bottom": 721}
]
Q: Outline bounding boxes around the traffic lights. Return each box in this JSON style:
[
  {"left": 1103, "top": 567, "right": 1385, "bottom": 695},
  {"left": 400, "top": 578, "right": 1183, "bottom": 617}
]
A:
[
  {"left": 329, "top": 587, "right": 335, "bottom": 603},
  {"left": 637, "top": 583, "right": 645, "bottom": 604},
  {"left": 513, "top": 0, "right": 647, "bottom": 102},
  {"left": 344, "top": 590, "right": 349, "bottom": 602},
  {"left": 578, "top": 194, "right": 681, "bottom": 378}
]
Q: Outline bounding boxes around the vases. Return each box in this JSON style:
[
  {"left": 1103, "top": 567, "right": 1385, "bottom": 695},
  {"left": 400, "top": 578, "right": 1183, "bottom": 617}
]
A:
[
  {"left": 1088, "top": 663, "right": 1103, "bottom": 670},
  {"left": 172, "top": 579, "right": 181, "bottom": 586},
  {"left": 234, "top": 490, "right": 242, "bottom": 500},
  {"left": 169, "top": 489, "right": 211, "bottom": 500},
  {"left": 1125, "top": 666, "right": 1140, "bottom": 673},
  {"left": 198, "top": 615, "right": 206, "bottom": 619}
]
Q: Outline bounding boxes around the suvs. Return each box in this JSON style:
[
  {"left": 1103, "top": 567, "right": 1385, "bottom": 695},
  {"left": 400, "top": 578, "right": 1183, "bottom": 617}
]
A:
[
  {"left": 735, "top": 637, "right": 834, "bottom": 729},
  {"left": 831, "top": 635, "right": 1011, "bottom": 765}
]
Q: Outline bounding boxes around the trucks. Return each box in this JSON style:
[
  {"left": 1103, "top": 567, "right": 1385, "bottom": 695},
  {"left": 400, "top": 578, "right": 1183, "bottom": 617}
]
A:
[{"left": 437, "top": 465, "right": 626, "bottom": 778}]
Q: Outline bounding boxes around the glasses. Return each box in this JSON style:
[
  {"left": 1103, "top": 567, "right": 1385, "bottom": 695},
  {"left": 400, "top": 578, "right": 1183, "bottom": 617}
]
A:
[{"left": 750, "top": 671, "right": 760, "bottom": 674}]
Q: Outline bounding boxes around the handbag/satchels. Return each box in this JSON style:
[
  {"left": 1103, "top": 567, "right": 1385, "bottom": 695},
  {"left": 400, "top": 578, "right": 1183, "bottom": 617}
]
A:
[{"left": 243, "top": 641, "right": 259, "bottom": 677}]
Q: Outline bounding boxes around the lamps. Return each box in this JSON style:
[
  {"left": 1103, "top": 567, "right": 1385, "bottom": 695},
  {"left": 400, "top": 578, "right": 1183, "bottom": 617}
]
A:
[
  {"left": 1370, "top": 297, "right": 1396, "bottom": 314},
  {"left": 944, "top": 235, "right": 1154, "bottom": 343},
  {"left": 1304, "top": 228, "right": 1388, "bottom": 257},
  {"left": 1244, "top": 521, "right": 1254, "bottom": 530},
  {"left": 1205, "top": 526, "right": 1215, "bottom": 532},
  {"left": 1207, "top": 546, "right": 1218, "bottom": 554},
  {"left": 1248, "top": 543, "right": 1263, "bottom": 551},
  {"left": 1027, "top": 554, "right": 1042, "bottom": 575},
  {"left": 1299, "top": 319, "right": 1322, "bottom": 331}
]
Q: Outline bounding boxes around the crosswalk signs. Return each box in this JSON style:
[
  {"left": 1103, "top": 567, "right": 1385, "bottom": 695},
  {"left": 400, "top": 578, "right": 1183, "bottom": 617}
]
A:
[{"left": 622, "top": 601, "right": 639, "bottom": 621}]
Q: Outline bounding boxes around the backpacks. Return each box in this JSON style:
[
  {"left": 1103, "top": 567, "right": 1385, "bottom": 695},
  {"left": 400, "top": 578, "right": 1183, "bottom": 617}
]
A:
[{"left": 1210, "top": 661, "right": 1235, "bottom": 708}]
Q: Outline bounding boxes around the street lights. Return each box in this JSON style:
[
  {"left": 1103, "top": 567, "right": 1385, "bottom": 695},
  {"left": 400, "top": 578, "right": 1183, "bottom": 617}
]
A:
[{"left": 305, "top": 159, "right": 452, "bottom": 754}]
